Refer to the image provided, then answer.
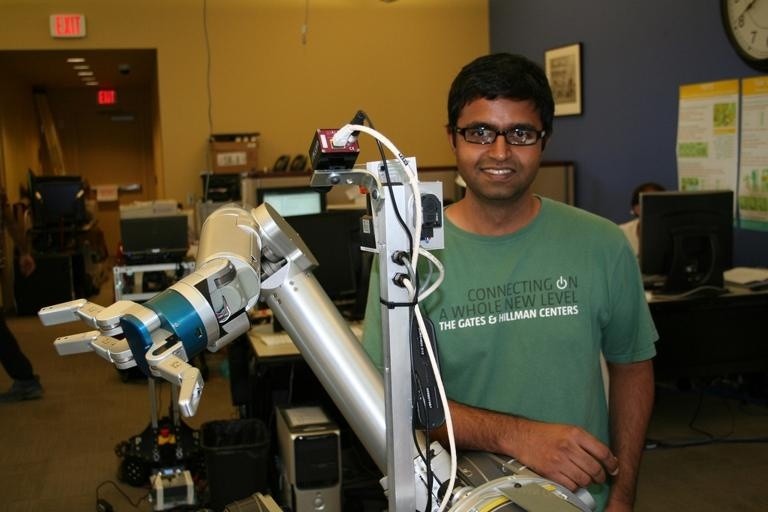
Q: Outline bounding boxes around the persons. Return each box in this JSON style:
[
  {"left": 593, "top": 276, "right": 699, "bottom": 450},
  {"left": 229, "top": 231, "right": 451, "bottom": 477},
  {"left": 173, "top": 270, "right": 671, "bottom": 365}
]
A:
[
  {"left": 1, "top": 183, "right": 47, "bottom": 404},
  {"left": 362, "top": 51, "right": 662, "bottom": 510},
  {"left": 615, "top": 183, "right": 666, "bottom": 257}
]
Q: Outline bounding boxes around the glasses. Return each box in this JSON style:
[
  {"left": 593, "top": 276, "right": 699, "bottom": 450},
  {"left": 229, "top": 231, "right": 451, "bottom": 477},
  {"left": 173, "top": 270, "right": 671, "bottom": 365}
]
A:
[{"left": 451, "top": 124, "right": 546, "bottom": 145}]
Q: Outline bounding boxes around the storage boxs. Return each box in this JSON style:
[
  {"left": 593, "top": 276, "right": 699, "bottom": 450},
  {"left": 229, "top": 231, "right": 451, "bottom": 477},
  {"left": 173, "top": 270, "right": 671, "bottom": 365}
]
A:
[{"left": 209, "top": 141, "right": 257, "bottom": 174}]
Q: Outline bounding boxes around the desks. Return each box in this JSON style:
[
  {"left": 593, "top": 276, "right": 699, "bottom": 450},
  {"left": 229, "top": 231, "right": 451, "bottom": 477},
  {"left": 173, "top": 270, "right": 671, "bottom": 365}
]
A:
[{"left": 230, "top": 267, "right": 768, "bottom": 436}]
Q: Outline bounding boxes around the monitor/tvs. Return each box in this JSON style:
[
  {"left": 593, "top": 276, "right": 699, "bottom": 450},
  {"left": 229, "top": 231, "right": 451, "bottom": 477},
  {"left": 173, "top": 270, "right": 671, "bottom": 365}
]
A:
[
  {"left": 274, "top": 210, "right": 380, "bottom": 332},
  {"left": 257, "top": 185, "right": 329, "bottom": 221},
  {"left": 638, "top": 188, "right": 738, "bottom": 297}
]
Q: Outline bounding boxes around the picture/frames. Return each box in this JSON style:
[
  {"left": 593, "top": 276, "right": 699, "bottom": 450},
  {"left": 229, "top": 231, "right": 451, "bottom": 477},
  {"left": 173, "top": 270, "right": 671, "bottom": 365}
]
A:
[{"left": 543, "top": 42, "right": 583, "bottom": 118}]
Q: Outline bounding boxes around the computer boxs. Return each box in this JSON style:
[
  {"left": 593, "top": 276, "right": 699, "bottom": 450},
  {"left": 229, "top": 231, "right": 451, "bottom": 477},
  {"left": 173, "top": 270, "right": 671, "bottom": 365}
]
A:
[{"left": 275, "top": 404, "right": 342, "bottom": 511}]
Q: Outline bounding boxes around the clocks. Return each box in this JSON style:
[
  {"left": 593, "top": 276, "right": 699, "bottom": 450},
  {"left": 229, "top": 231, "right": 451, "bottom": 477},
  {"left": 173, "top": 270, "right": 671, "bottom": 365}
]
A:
[{"left": 718, "top": 0, "right": 768, "bottom": 74}]
